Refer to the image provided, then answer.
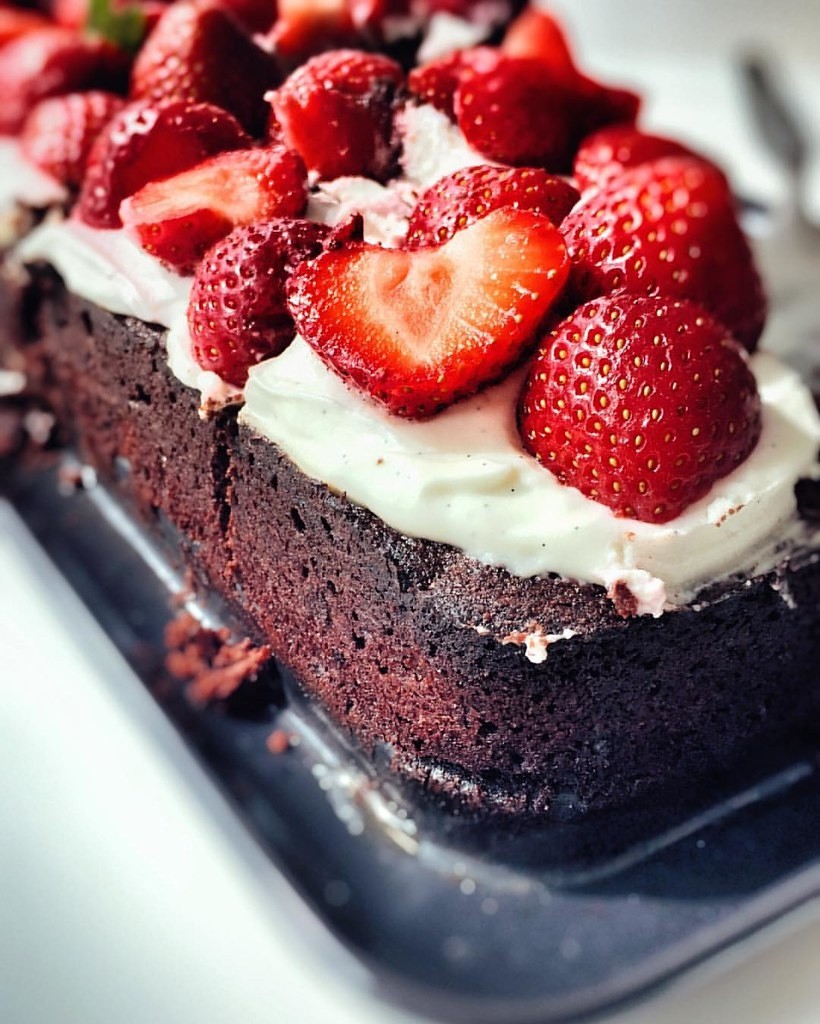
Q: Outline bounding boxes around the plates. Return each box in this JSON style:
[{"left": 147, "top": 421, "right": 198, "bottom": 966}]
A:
[{"left": 1, "top": 376, "right": 818, "bottom": 1023}]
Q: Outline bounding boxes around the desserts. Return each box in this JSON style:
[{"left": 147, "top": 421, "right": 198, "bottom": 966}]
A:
[{"left": 1, "top": 1, "right": 819, "bottom": 816}]
